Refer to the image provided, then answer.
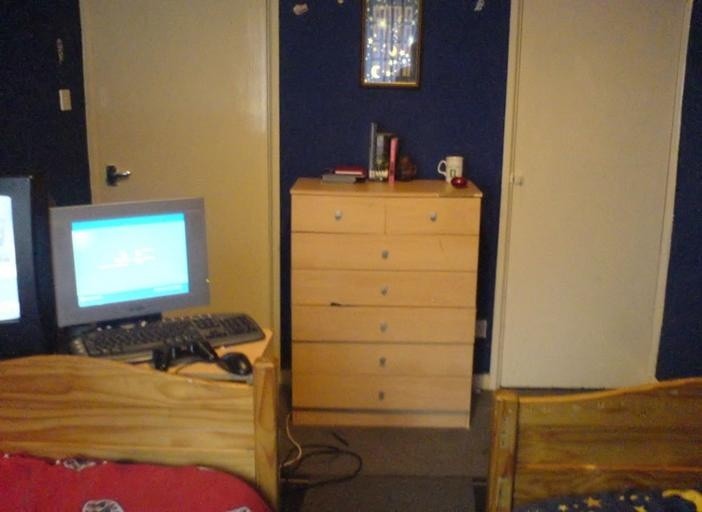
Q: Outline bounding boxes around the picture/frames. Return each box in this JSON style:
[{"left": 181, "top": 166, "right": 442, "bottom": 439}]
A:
[{"left": 359, "top": 0, "right": 425, "bottom": 93}]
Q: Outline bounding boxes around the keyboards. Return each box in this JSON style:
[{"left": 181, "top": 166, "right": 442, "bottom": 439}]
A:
[{"left": 65, "top": 311, "right": 265, "bottom": 362}]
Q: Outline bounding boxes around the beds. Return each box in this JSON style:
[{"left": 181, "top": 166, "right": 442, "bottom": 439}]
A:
[
  {"left": 487, "top": 378, "right": 701, "bottom": 512},
  {"left": 0, "top": 357, "right": 280, "bottom": 511}
]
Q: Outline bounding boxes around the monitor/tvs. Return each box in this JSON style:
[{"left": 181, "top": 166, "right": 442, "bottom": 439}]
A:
[
  {"left": 48, "top": 196, "right": 211, "bottom": 330},
  {"left": 0, "top": 176, "right": 44, "bottom": 346}
]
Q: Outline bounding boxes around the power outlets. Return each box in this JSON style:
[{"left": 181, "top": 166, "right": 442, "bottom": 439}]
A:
[{"left": 476, "top": 321, "right": 487, "bottom": 338}]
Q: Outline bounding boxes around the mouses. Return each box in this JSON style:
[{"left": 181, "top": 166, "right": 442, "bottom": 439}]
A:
[{"left": 219, "top": 349, "right": 251, "bottom": 376}]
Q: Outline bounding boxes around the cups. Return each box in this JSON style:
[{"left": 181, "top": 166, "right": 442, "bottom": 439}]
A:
[{"left": 437, "top": 156, "right": 464, "bottom": 183}]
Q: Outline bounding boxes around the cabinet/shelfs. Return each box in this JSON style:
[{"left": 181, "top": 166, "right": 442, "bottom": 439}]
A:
[{"left": 286, "top": 175, "right": 485, "bottom": 429}]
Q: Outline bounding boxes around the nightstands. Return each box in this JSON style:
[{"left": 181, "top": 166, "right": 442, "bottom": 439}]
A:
[{"left": 128, "top": 329, "right": 274, "bottom": 380}]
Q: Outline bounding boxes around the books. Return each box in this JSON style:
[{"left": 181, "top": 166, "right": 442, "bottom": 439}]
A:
[
  {"left": 321, "top": 173, "right": 358, "bottom": 184},
  {"left": 375, "top": 130, "right": 391, "bottom": 182},
  {"left": 368, "top": 122, "right": 379, "bottom": 180},
  {"left": 389, "top": 135, "right": 401, "bottom": 182},
  {"left": 333, "top": 165, "right": 366, "bottom": 175}
]
